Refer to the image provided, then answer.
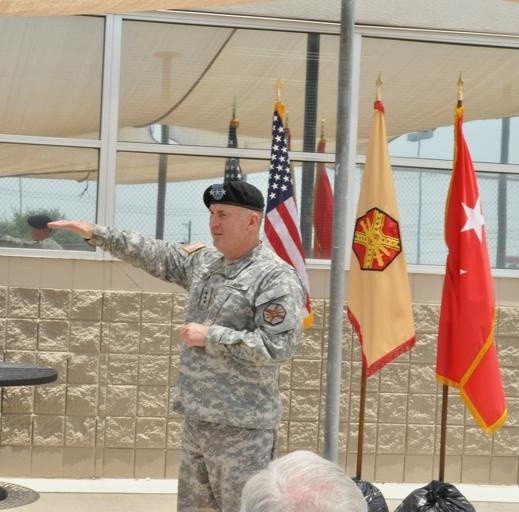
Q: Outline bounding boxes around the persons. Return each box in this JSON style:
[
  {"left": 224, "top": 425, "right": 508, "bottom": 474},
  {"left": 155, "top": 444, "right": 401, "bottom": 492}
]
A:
[
  {"left": 46, "top": 180, "right": 306, "bottom": 511},
  {"left": 0, "top": 215, "right": 61, "bottom": 249},
  {"left": 239, "top": 450, "right": 369, "bottom": 511}
]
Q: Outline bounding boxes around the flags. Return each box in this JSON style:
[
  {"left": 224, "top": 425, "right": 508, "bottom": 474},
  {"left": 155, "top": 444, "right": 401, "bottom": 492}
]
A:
[
  {"left": 434, "top": 89, "right": 509, "bottom": 436},
  {"left": 224, "top": 119, "right": 246, "bottom": 183},
  {"left": 312, "top": 137, "right": 335, "bottom": 260},
  {"left": 262, "top": 99, "right": 315, "bottom": 334},
  {"left": 346, "top": 99, "right": 418, "bottom": 378}
]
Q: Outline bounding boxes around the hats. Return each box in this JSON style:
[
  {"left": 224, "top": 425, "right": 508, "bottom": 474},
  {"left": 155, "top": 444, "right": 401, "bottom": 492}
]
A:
[
  {"left": 203, "top": 180, "right": 265, "bottom": 212},
  {"left": 27, "top": 215, "right": 52, "bottom": 228}
]
